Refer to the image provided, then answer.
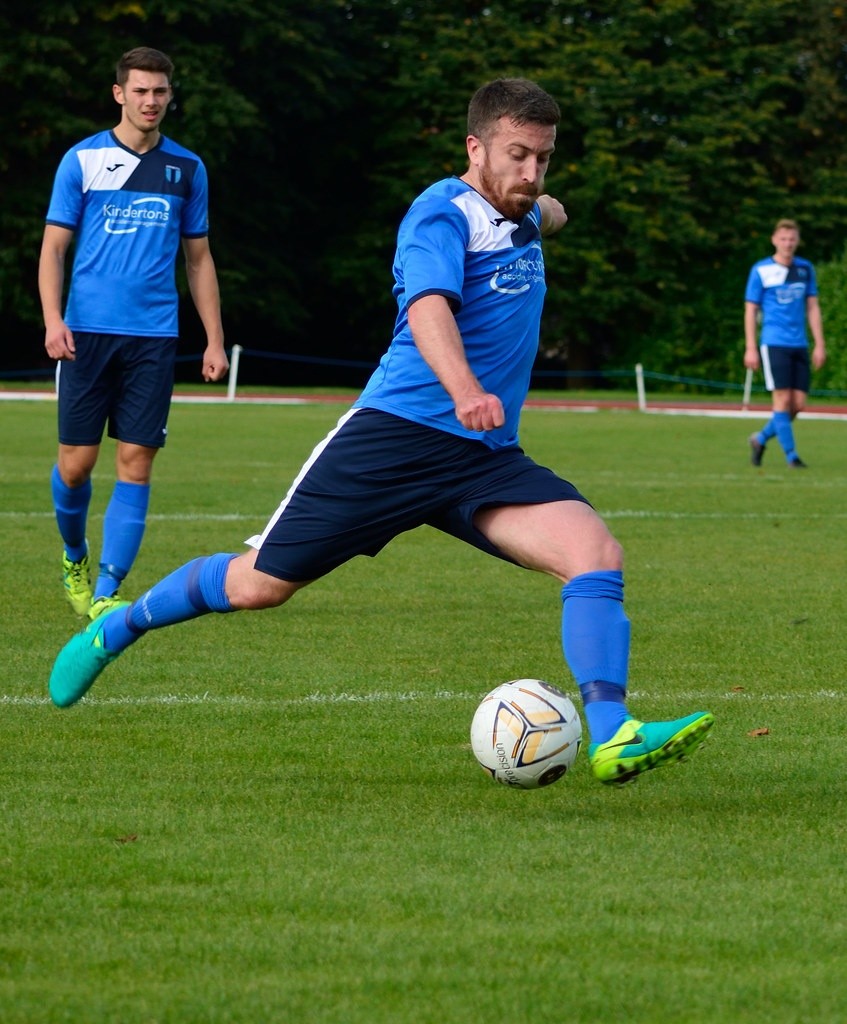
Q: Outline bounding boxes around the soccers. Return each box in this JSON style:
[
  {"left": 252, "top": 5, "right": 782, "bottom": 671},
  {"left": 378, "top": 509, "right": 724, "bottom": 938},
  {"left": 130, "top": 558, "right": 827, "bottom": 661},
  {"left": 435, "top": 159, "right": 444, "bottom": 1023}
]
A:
[{"left": 471, "top": 679, "right": 582, "bottom": 790}]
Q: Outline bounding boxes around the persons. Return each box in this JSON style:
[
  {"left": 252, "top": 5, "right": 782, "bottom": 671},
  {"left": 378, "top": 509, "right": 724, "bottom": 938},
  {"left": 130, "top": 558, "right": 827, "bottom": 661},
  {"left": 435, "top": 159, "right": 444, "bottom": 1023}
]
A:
[
  {"left": 44, "top": 74, "right": 714, "bottom": 785},
  {"left": 743, "top": 216, "right": 827, "bottom": 469},
  {"left": 37, "top": 44, "right": 229, "bottom": 622}
]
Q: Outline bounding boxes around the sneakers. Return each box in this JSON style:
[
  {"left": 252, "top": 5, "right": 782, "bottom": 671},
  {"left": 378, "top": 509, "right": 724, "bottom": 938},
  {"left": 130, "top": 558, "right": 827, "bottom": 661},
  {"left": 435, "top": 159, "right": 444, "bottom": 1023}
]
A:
[
  {"left": 88, "top": 595, "right": 135, "bottom": 623},
  {"left": 786, "top": 457, "right": 805, "bottom": 472},
  {"left": 50, "top": 602, "right": 135, "bottom": 709},
  {"left": 59, "top": 538, "right": 92, "bottom": 617},
  {"left": 749, "top": 428, "right": 766, "bottom": 467},
  {"left": 591, "top": 710, "right": 714, "bottom": 783}
]
